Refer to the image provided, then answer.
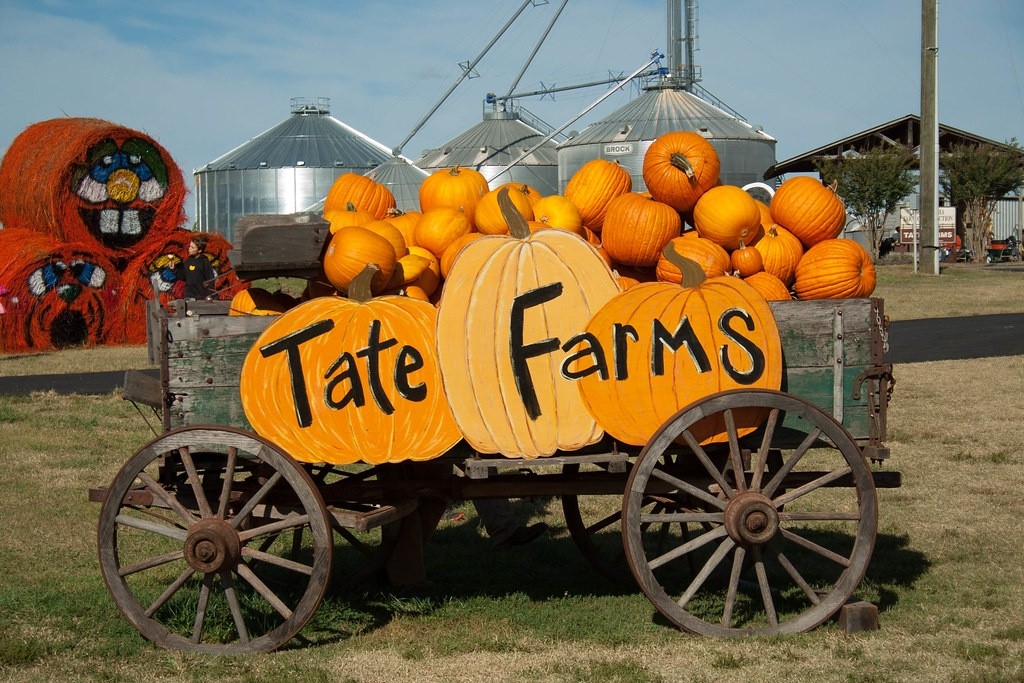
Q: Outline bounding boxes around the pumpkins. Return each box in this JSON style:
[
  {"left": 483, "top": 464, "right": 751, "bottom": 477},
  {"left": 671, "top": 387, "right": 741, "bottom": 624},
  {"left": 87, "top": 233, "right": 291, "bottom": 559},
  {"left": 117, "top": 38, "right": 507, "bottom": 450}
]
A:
[{"left": 228, "top": 134, "right": 877, "bottom": 316}]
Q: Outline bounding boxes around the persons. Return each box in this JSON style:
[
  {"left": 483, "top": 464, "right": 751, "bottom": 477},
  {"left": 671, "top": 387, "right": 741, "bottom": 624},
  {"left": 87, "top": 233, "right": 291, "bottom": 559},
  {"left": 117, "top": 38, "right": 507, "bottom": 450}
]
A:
[
  {"left": 369, "top": 502, "right": 445, "bottom": 597},
  {"left": 472, "top": 494, "right": 548, "bottom": 551},
  {"left": 169, "top": 236, "right": 215, "bottom": 301}
]
